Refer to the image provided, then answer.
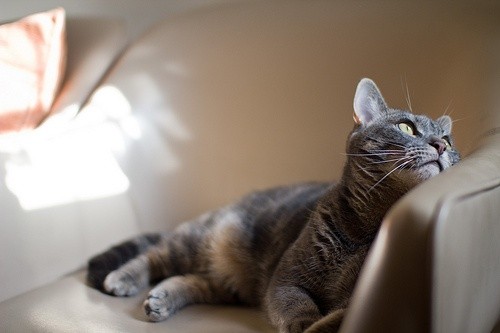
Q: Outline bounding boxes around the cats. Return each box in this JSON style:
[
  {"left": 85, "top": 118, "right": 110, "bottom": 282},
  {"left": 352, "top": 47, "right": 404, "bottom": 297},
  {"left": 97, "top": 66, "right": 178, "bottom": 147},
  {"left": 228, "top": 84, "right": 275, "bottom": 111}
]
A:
[{"left": 87, "top": 77, "right": 463, "bottom": 333}]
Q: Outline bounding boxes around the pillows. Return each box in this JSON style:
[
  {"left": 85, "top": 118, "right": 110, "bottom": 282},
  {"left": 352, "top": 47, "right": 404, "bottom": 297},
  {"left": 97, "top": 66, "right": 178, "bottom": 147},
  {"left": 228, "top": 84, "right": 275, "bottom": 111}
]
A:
[{"left": 0, "top": 6, "right": 69, "bottom": 133}]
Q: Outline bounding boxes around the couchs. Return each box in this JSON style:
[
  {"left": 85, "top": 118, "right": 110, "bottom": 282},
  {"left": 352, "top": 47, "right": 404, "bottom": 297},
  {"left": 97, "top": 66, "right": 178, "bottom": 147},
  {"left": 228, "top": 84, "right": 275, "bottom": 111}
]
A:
[
  {"left": 2, "top": 1, "right": 500, "bottom": 333},
  {"left": 1, "top": 16, "right": 128, "bottom": 143}
]
